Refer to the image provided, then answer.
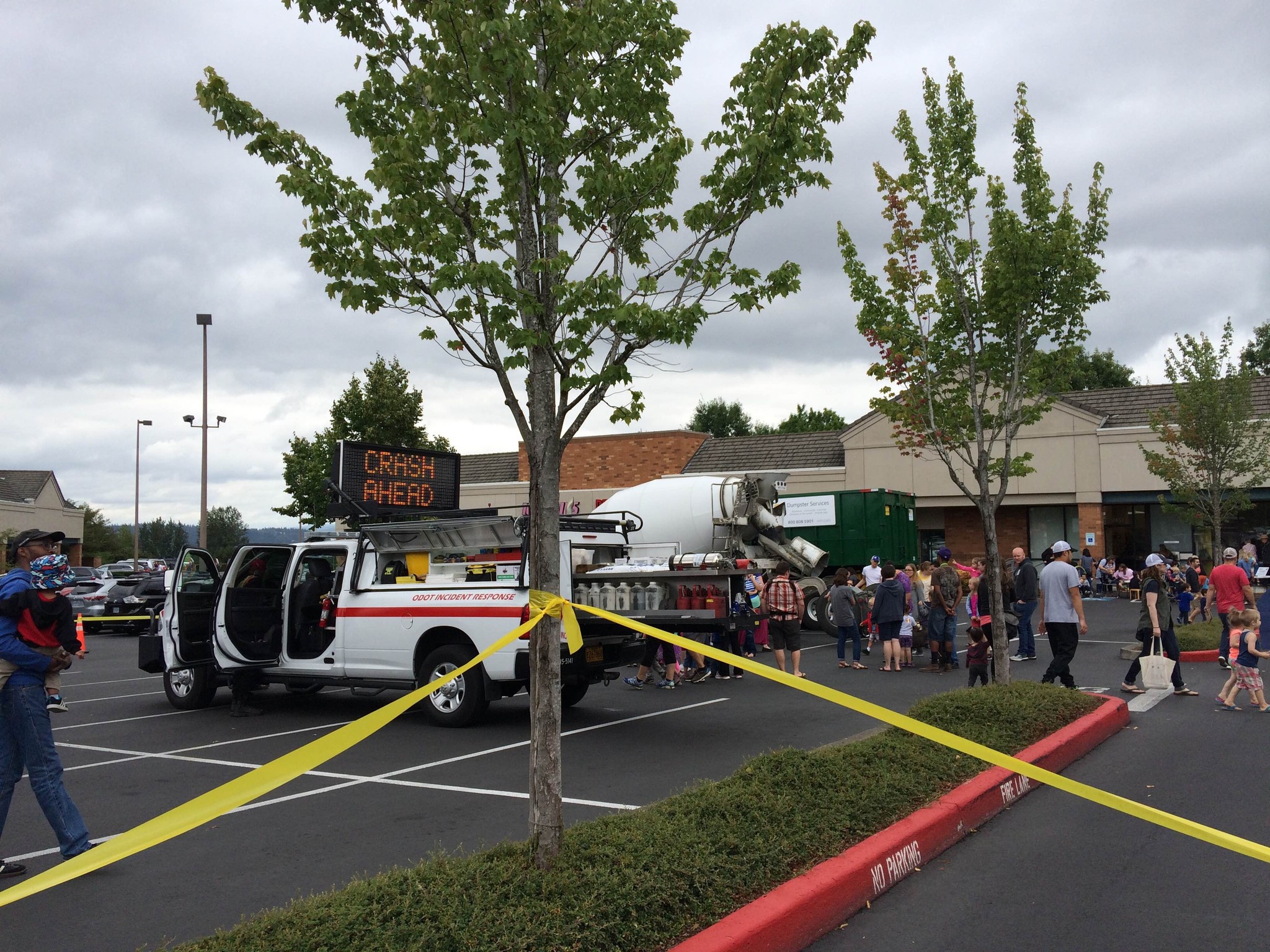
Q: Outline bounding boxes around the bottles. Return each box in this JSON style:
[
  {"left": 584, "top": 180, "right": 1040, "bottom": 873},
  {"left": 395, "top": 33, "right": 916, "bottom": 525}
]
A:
[
  {"left": 600, "top": 583, "right": 616, "bottom": 610},
  {"left": 645, "top": 582, "right": 669, "bottom": 610},
  {"left": 630, "top": 582, "right": 646, "bottom": 610},
  {"left": 573, "top": 583, "right": 589, "bottom": 610},
  {"left": 587, "top": 583, "right": 600, "bottom": 609},
  {"left": 615, "top": 582, "right": 633, "bottom": 610}
]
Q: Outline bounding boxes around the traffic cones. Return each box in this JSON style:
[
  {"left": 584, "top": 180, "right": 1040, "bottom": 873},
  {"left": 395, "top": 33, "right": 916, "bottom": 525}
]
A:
[{"left": 76, "top": 614, "right": 90, "bottom": 653}]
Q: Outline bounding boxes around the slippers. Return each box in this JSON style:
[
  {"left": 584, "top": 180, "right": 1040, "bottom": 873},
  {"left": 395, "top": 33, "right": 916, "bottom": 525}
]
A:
[
  {"left": 878, "top": 666, "right": 903, "bottom": 672},
  {"left": 1214, "top": 655, "right": 1270, "bottom": 713},
  {"left": 1119, "top": 687, "right": 1201, "bottom": 696}
]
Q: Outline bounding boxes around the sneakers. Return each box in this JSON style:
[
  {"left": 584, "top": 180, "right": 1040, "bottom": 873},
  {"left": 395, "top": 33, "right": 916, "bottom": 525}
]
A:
[{"left": 623, "top": 663, "right": 743, "bottom": 690}]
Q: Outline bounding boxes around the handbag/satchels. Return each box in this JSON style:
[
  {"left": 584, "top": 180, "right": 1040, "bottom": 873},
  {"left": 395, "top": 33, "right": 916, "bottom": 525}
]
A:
[{"left": 1138, "top": 634, "right": 1176, "bottom": 691}]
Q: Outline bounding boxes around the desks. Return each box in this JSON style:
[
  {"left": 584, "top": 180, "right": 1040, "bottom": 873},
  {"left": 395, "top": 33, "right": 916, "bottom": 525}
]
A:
[
  {"left": 1124, "top": 580, "right": 1143, "bottom": 598},
  {"left": 1253, "top": 575, "right": 1270, "bottom": 594}
]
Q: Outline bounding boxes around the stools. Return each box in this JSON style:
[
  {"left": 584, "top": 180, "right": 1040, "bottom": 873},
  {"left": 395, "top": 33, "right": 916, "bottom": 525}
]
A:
[{"left": 1130, "top": 589, "right": 1141, "bottom": 600}]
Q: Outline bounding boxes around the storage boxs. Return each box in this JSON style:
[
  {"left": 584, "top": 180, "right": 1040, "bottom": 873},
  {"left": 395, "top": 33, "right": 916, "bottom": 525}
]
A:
[
  {"left": 395, "top": 576, "right": 425, "bottom": 584},
  {"left": 615, "top": 557, "right": 668, "bottom": 566},
  {"left": 576, "top": 564, "right": 609, "bottom": 573},
  {"left": 496, "top": 566, "right": 521, "bottom": 581}
]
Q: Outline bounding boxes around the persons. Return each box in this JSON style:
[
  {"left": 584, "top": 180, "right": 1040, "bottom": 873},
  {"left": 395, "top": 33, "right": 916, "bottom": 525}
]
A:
[
  {"left": 1041, "top": 543, "right": 1146, "bottom": 599},
  {"left": 919, "top": 547, "right": 963, "bottom": 672},
  {"left": 1158, "top": 544, "right": 1213, "bottom": 624},
  {"left": 760, "top": 561, "right": 806, "bottom": 679},
  {"left": 0, "top": 529, "right": 106, "bottom": 877},
  {"left": 929, "top": 595, "right": 959, "bottom": 670},
  {"left": 950, "top": 556, "right": 1016, "bottom": 689},
  {"left": 1120, "top": 554, "right": 1200, "bottom": 696},
  {"left": 1009, "top": 547, "right": 1038, "bottom": 661},
  {"left": 1037, "top": 540, "right": 1088, "bottom": 691},
  {"left": 824, "top": 548, "right": 940, "bottom": 672},
  {"left": 1215, "top": 532, "right": 1270, "bottom": 713},
  {"left": 622, "top": 574, "right": 773, "bottom": 689},
  {"left": 1204, "top": 547, "right": 1257, "bottom": 669}
]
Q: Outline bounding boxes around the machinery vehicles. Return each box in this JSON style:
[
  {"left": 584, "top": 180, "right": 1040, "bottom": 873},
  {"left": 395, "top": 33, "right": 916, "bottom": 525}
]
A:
[{"left": 580, "top": 472, "right": 832, "bottom": 585}]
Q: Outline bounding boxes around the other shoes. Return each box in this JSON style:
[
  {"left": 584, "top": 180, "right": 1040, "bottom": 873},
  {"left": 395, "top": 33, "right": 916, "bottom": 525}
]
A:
[
  {"left": 1176, "top": 616, "right": 1193, "bottom": 625},
  {"left": 1009, "top": 651, "right": 1037, "bottom": 661},
  {"left": 799, "top": 672, "right": 806, "bottom": 678},
  {"left": 44, "top": 695, "right": 70, "bottom": 714},
  {"left": 61, "top": 842, "right": 102, "bottom": 862},
  {"left": 0, "top": 858, "right": 28, "bottom": 878},
  {"left": 861, "top": 648, "right": 871, "bottom": 655},
  {"left": 762, "top": 646, "right": 774, "bottom": 652},
  {"left": 882, "top": 649, "right": 960, "bottom": 673}
]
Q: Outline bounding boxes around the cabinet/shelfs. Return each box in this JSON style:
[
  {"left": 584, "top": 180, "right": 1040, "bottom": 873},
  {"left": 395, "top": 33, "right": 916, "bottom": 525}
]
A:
[{"left": 475, "top": 552, "right": 522, "bottom": 561}]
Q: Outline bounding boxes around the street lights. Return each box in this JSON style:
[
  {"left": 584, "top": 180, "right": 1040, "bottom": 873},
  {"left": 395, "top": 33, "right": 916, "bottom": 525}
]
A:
[
  {"left": 134, "top": 419, "right": 153, "bottom": 571},
  {"left": 183, "top": 314, "right": 227, "bottom": 573}
]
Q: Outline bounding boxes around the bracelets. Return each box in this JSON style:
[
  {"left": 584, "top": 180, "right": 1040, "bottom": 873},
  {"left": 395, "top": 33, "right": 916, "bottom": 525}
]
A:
[{"left": 1153, "top": 627, "right": 1160, "bottom": 628}]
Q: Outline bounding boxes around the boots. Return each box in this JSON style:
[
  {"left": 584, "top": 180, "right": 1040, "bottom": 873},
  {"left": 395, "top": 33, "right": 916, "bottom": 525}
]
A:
[{"left": 228, "top": 690, "right": 265, "bottom": 718}]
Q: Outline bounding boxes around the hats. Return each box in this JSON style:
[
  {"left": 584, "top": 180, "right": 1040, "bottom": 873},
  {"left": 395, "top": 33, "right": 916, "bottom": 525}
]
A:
[
  {"left": 1260, "top": 534, "right": 1269, "bottom": 539},
  {"left": 937, "top": 548, "right": 953, "bottom": 561},
  {"left": 27, "top": 552, "right": 76, "bottom": 590},
  {"left": 9, "top": 529, "right": 66, "bottom": 558},
  {"left": 1145, "top": 553, "right": 1174, "bottom": 568},
  {"left": 870, "top": 555, "right": 880, "bottom": 562},
  {"left": 1052, "top": 540, "right": 1078, "bottom": 555},
  {"left": 1222, "top": 547, "right": 1237, "bottom": 559}
]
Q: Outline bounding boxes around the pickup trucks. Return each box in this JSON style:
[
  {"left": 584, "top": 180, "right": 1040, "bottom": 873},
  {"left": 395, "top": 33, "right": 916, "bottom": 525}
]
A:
[{"left": 140, "top": 505, "right": 647, "bottom": 728}]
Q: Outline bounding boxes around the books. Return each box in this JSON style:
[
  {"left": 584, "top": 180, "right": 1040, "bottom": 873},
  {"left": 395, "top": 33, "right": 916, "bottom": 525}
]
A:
[{"left": 1204, "top": 609, "right": 1213, "bottom": 624}]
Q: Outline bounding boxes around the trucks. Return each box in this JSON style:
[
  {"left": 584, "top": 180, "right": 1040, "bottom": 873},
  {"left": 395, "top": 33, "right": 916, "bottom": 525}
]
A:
[{"left": 772, "top": 488, "right": 919, "bottom": 630}]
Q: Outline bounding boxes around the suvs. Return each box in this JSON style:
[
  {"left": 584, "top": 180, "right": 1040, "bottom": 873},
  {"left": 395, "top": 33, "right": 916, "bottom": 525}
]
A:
[{"left": 0, "top": 559, "right": 227, "bottom": 635}]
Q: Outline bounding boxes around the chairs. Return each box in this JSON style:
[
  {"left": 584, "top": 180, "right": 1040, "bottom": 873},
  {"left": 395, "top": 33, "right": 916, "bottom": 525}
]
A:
[
  {"left": 1101, "top": 572, "right": 1116, "bottom": 596},
  {"left": 1199, "top": 575, "right": 1207, "bottom": 590},
  {"left": 293, "top": 558, "right": 333, "bottom": 652}
]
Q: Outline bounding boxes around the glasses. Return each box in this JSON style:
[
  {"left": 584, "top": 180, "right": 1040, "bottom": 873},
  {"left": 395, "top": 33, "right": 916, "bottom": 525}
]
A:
[
  {"left": 19, "top": 541, "right": 60, "bottom": 552},
  {"left": 904, "top": 569, "right": 914, "bottom": 572}
]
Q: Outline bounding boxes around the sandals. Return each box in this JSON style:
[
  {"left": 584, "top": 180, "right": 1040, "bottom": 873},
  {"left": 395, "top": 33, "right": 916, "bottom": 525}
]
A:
[{"left": 839, "top": 661, "right": 869, "bottom": 669}]
